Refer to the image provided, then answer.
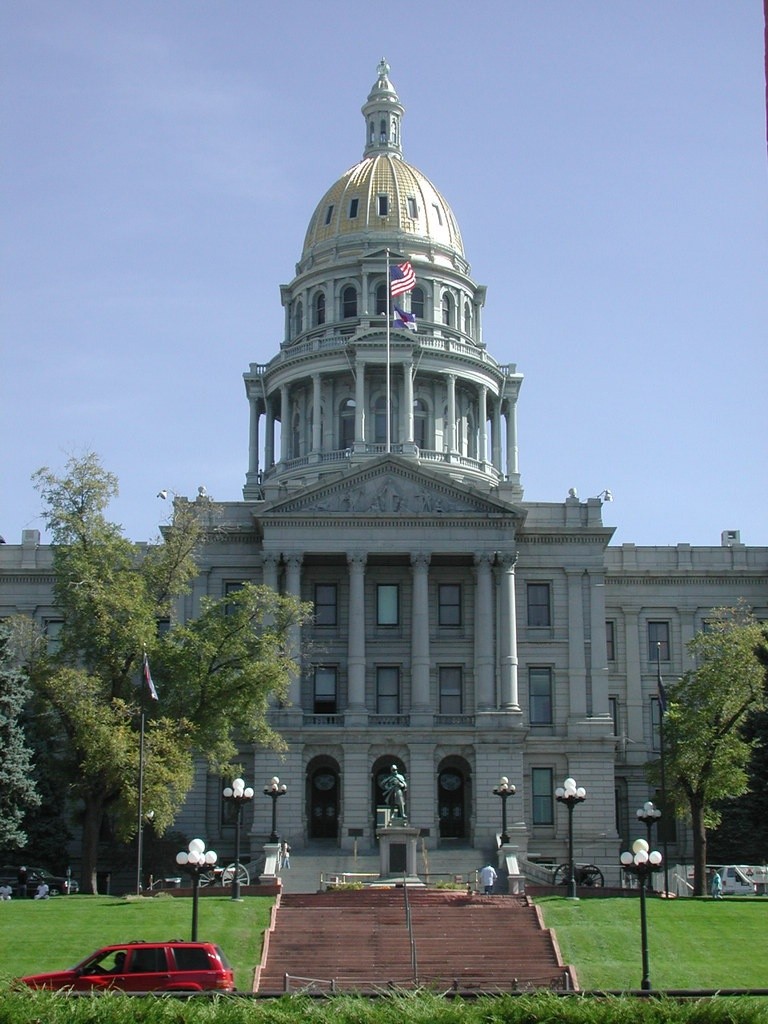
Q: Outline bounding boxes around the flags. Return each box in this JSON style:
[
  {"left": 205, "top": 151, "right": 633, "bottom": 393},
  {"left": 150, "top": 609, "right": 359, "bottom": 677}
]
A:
[
  {"left": 658, "top": 678, "right": 667, "bottom": 714},
  {"left": 393, "top": 305, "right": 417, "bottom": 331},
  {"left": 389, "top": 261, "right": 416, "bottom": 297},
  {"left": 145, "top": 659, "right": 158, "bottom": 700}
]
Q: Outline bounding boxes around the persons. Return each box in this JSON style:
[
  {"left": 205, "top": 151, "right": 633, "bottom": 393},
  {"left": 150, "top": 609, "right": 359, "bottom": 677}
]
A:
[
  {"left": 711, "top": 869, "right": 722, "bottom": 899},
  {"left": 17, "top": 867, "right": 27, "bottom": 897},
  {"left": 0, "top": 880, "right": 12, "bottom": 900},
  {"left": 281, "top": 840, "right": 290, "bottom": 869},
  {"left": 480, "top": 863, "right": 497, "bottom": 894},
  {"left": 380, "top": 765, "right": 407, "bottom": 819},
  {"left": 107, "top": 952, "right": 126, "bottom": 974},
  {"left": 34, "top": 881, "right": 50, "bottom": 899},
  {"left": 380, "top": 311, "right": 385, "bottom": 315}
]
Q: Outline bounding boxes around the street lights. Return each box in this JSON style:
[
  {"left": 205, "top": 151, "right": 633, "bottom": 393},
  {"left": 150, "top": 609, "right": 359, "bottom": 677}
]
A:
[
  {"left": 262, "top": 775, "right": 287, "bottom": 842},
  {"left": 619, "top": 838, "right": 663, "bottom": 989},
  {"left": 553, "top": 777, "right": 587, "bottom": 901},
  {"left": 174, "top": 837, "right": 219, "bottom": 940},
  {"left": 635, "top": 800, "right": 663, "bottom": 889},
  {"left": 492, "top": 777, "right": 516, "bottom": 849},
  {"left": 221, "top": 777, "right": 256, "bottom": 901}
]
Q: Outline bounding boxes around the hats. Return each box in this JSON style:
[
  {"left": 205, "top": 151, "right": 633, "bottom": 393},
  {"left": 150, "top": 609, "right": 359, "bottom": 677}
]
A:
[{"left": 20, "top": 866, "right": 26, "bottom": 871}]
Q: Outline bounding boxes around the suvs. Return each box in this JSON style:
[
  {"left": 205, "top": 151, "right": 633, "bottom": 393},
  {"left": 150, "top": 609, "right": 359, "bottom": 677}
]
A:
[
  {"left": 11, "top": 939, "right": 239, "bottom": 1001},
  {"left": 0, "top": 866, "right": 79, "bottom": 896}
]
